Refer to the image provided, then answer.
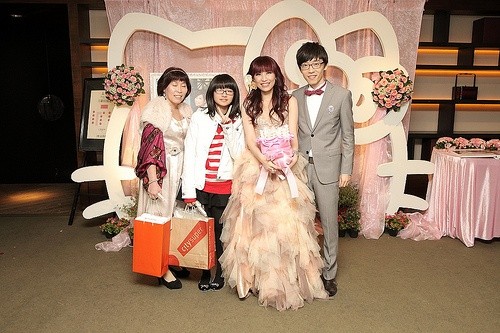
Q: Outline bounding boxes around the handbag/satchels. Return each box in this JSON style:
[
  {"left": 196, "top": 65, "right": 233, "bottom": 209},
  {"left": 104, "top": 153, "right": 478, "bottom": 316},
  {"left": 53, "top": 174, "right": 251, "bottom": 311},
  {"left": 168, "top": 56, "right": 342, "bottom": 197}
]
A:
[
  {"left": 168, "top": 204, "right": 215, "bottom": 270},
  {"left": 132, "top": 197, "right": 171, "bottom": 278}
]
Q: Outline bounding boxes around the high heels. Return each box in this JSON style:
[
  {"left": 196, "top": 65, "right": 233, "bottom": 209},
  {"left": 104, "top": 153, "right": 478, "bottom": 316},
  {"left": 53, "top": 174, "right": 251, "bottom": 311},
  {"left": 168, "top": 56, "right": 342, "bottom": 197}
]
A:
[
  {"left": 169, "top": 266, "right": 190, "bottom": 277},
  {"left": 157, "top": 269, "right": 182, "bottom": 289}
]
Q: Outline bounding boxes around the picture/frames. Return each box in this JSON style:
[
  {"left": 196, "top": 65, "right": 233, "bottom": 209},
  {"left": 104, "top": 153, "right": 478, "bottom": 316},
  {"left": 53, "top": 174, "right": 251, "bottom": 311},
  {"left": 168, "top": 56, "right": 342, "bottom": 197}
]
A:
[{"left": 150, "top": 73, "right": 225, "bottom": 114}]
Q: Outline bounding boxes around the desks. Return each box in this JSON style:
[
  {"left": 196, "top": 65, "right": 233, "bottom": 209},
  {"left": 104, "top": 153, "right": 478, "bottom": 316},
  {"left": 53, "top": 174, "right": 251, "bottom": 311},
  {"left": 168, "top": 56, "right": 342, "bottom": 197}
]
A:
[{"left": 423, "top": 145, "right": 500, "bottom": 247}]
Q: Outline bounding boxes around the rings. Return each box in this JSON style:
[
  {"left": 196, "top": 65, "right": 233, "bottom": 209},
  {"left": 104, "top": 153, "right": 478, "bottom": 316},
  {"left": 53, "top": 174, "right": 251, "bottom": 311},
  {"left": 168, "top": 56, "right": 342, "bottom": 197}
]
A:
[{"left": 269, "top": 167, "right": 274, "bottom": 171}]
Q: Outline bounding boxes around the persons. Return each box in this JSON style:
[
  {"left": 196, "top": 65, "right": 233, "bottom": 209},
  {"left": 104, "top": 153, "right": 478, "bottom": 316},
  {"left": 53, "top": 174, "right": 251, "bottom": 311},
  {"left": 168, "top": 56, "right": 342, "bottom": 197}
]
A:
[
  {"left": 218, "top": 56, "right": 330, "bottom": 311},
  {"left": 191, "top": 90, "right": 205, "bottom": 107},
  {"left": 291, "top": 42, "right": 353, "bottom": 295},
  {"left": 135, "top": 66, "right": 195, "bottom": 289},
  {"left": 181, "top": 74, "right": 241, "bottom": 291}
]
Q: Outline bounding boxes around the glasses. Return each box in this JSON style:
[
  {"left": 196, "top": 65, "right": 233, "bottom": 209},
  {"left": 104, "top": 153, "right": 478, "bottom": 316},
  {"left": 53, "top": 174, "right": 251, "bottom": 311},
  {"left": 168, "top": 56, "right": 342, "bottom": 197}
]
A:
[
  {"left": 215, "top": 90, "right": 234, "bottom": 95},
  {"left": 299, "top": 62, "right": 324, "bottom": 69}
]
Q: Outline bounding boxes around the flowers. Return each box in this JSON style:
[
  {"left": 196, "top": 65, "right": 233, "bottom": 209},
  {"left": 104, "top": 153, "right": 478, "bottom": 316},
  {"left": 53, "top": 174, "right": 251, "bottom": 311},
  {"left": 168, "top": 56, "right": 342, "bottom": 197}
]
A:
[
  {"left": 99, "top": 190, "right": 139, "bottom": 239},
  {"left": 371, "top": 68, "right": 414, "bottom": 113},
  {"left": 103, "top": 65, "right": 146, "bottom": 106},
  {"left": 436, "top": 137, "right": 500, "bottom": 152},
  {"left": 258, "top": 126, "right": 295, "bottom": 166},
  {"left": 245, "top": 74, "right": 258, "bottom": 91},
  {"left": 384, "top": 211, "right": 410, "bottom": 231}
]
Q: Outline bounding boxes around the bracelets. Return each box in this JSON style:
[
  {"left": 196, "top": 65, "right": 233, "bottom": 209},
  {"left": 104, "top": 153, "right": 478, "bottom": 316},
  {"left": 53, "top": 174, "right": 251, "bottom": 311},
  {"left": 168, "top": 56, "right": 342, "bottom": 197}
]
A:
[{"left": 292, "top": 150, "right": 299, "bottom": 156}]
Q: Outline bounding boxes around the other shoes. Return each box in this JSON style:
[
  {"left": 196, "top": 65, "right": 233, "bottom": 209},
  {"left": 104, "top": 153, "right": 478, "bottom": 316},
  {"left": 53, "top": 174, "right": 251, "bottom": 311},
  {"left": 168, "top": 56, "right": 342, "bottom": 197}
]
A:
[
  {"left": 198, "top": 274, "right": 210, "bottom": 291},
  {"left": 210, "top": 277, "right": 225, "bottom": 290},
  {"left": 321, "top": 276, "right": 338, "bottom": 298}
]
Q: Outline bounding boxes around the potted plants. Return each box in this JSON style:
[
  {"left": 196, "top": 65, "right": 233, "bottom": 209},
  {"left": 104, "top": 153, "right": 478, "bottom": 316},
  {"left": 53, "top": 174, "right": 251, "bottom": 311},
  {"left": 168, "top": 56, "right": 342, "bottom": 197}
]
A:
[{"left": 338, "top": 184, "right": 361, "bottom": 239}]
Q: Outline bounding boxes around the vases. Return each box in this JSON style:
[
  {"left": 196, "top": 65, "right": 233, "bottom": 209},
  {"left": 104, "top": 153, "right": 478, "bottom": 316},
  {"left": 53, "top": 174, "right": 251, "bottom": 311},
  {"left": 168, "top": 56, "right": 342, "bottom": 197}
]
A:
[{"left": 389, "top": 228, "right": 398, "bottom": 238}]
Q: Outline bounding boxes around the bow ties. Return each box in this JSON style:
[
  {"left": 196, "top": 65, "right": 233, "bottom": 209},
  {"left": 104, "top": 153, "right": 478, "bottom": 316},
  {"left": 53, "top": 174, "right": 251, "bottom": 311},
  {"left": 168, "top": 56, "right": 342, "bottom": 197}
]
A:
[{"left": 304, "top": 82, "right": 326, "bottom": 96}]
